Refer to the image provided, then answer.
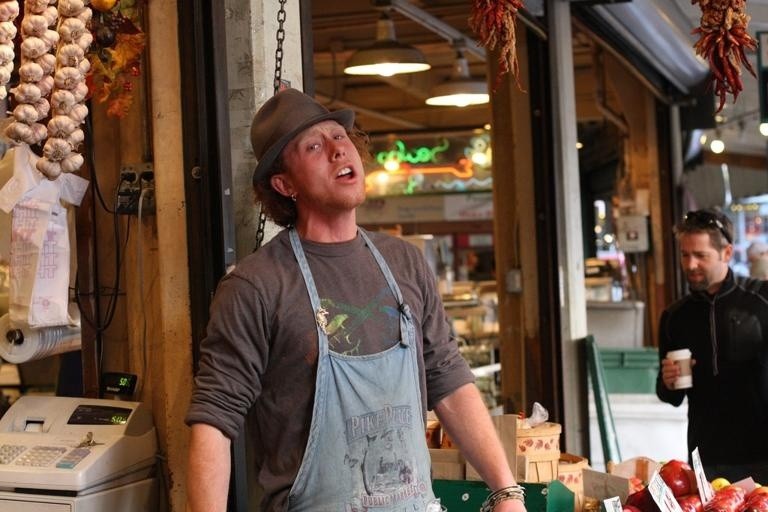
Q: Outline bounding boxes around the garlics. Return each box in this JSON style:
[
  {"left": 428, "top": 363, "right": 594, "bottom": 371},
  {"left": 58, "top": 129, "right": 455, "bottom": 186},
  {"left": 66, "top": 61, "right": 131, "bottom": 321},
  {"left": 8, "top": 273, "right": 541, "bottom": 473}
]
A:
[{"left": 0, "top": 0, "right": 94, "bottom": 181}]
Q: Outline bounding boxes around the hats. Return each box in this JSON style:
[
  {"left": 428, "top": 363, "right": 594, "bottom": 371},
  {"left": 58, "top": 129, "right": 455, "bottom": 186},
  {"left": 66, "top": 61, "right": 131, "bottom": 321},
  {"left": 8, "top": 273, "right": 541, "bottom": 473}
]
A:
[{"left": 251, "top": 88, "right": 355, "bottom": 190}]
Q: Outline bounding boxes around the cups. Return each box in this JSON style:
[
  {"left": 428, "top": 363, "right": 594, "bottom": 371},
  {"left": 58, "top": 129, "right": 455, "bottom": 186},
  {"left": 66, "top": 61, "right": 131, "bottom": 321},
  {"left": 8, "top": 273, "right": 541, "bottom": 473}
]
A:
[{"left": 665, "top": 348, "right": 694, "bottom": 391}]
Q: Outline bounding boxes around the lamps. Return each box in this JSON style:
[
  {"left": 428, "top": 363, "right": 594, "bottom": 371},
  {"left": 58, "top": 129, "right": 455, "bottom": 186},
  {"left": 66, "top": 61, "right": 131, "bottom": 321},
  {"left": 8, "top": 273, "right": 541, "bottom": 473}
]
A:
[{"left": 342, "top": 0, "right": 491, "bottom": 107}]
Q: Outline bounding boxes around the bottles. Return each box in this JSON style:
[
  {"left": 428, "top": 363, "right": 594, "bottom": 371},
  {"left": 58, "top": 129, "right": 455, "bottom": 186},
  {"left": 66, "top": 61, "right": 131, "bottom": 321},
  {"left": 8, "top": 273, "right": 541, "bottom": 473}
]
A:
[
  {"left": 458, "top": 258, "right": 469, "bottom": 285},
  {"left": 611, "top": 280, "right": 622, "bottom": 303},
  {"left": 441, "top": 265, "right": 454, "bottom": 295}
]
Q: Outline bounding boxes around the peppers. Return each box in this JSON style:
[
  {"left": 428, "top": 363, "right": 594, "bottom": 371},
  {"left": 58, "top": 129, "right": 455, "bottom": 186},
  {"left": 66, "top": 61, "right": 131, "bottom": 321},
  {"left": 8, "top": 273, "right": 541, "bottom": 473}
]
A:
[
  {"left": 468, "top": 0, "right": 528, "bottom": 100},
  {"left": 690, "top": 0, "right": 760, "bottom": 116}
]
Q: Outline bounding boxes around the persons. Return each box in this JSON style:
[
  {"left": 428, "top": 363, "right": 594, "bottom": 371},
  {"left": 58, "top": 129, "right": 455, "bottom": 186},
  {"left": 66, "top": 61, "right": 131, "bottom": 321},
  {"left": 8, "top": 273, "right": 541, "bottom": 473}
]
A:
[
  {"left": 654, "top": 209, "right": 768, "bottom": 484},
  {"left": 182, "top": 86, "right": 533, "bottom": 512}
]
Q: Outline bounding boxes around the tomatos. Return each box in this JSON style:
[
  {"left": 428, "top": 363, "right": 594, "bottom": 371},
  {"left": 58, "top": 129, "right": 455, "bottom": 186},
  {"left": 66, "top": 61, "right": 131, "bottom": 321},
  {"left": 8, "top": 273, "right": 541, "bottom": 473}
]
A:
[{"left": 622, "top": 460, "right": 768, "bottom": 512}]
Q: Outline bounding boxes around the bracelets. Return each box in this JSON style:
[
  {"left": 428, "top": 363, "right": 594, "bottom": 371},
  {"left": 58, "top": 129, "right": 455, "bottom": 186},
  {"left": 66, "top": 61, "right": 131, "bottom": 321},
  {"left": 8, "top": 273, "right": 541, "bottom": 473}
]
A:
[{"left": 486, "top": 484, "right": 529, "bottom": 511}]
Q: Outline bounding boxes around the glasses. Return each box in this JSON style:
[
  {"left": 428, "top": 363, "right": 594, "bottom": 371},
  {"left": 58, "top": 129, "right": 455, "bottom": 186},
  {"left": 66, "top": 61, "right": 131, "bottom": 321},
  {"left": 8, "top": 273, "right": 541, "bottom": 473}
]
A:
[{"left": 683, "top": 210, "right": 732, "bottom": 243}]
